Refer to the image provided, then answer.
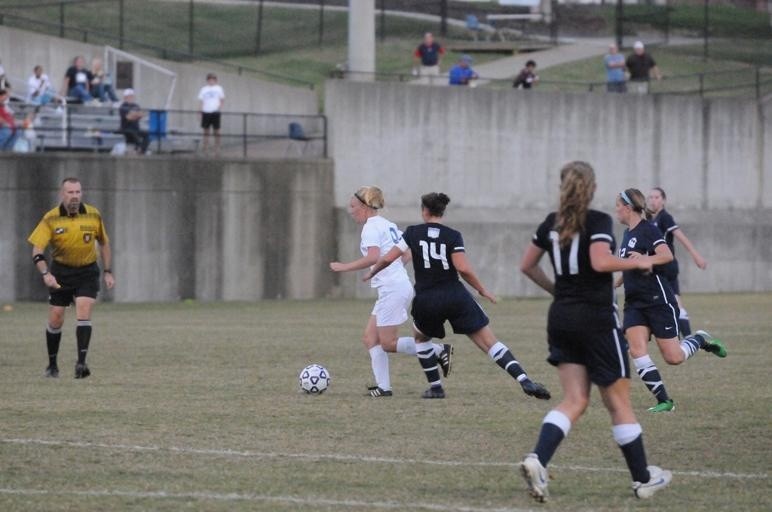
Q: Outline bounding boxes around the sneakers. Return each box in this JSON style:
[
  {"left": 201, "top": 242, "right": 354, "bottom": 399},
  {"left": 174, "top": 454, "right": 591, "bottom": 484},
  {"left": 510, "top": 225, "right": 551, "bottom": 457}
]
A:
[
  {"left": 629, "top": 464, "right": 673, "bottom": 500},
  {"left": 421, "top": 383, "right": 445, "bottom": 398},
  {"left": 519, "top": 378, "right": 552, "bottom": 400},
  {"left": 436, "top": 343, "right": 454, "bottom": 378},
  {"left": 73, "top": 362, "right": 91, "bottom": 379},
  {"left": 646, "top": 398, "right": 675, "bottom": 415},
  {"left": 518, "top": 452, "right": 551, "bottom": 503},
  {"left": 41, "top": 364, "right": 60, "bottom": 379},
  {"left": 363, "top": 386, "right": 393, "bottom": 397},
  {"left": 693, "top": 329, "right": 727, "bottom": 358}
]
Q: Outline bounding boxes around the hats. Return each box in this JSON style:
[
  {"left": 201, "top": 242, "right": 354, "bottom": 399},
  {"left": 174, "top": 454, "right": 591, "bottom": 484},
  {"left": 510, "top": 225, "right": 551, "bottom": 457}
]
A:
[
  {"left": 122, "top": 87, "right": 136, "bottom": 97},
  {"left": 633, "top": 40, "right": 644, "bottom": 49},
  {"left": 461, "top": 55, "right": 472, "bottom": 62}
]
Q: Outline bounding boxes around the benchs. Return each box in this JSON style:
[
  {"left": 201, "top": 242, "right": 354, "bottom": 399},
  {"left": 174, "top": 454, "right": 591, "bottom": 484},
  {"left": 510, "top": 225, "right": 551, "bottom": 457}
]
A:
[{"left": 1, "top": 87, "right": 172, "bottom": 155}]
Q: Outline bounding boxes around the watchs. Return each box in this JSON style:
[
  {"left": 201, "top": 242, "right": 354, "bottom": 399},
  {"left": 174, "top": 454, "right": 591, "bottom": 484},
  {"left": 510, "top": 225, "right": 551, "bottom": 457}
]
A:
[{"left": 40, "top": 269, "right": 50, "bottom": 276}]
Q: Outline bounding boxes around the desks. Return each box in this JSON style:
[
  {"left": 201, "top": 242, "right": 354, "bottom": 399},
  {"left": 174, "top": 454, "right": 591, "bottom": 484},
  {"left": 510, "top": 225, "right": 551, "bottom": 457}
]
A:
[{"left": 486, "top": 13, "right": 545, "bottom": 42}]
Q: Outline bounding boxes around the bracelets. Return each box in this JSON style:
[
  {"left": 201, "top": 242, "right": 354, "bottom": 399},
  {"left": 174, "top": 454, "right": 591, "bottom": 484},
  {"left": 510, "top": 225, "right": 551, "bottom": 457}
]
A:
[{"left": 104, "top": 268, "right": 112, "bottom": 273}]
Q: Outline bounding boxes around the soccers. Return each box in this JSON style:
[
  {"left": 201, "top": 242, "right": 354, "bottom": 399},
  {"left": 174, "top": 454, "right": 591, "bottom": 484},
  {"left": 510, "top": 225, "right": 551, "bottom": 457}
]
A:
[{"left": 299, "top": 363, "right": 329, "bottom": 395}]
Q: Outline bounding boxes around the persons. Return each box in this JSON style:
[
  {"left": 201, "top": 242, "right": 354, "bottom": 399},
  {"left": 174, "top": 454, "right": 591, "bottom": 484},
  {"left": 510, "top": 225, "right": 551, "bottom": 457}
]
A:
[
  {"left": 603, "top": 43, "right": 627, "bottom": 93},
  {"left": 195, "top": 72, "right": 225, "bottom": 158},
  {"left": 464, "top": 12, "right": 561, "bottom": 44},
  {"left": 647, "top": 187, "right": 707, "bottom": 339},
  {"left": 518, "top": 161, "right": 672, "bottom": 502},
  {"left": 625, "top": 41, "right": 662, "bottom": 94},
  {"left": 412, "top": 32, "right": 445, "bottom": 84},
  {"left": 27, "top": 177, "right": 115, "bottom": 378},
  {"left": 613, "top": 187, "right": 727, "bottom": 413},
  {"left": 511, "top": 60, "right": 539, "bottom": 89},
  {"left": 329, "top": 185, "right": 454, "bottom": 397},
  {"left": 361, "top": 192, "right": 552, "bottom": 400},
  {"left": 0, "top": 54, "right": 152, "bottom": 156},
  {"left": 448, "top": 55, "right": 478, "bottom": 87}
]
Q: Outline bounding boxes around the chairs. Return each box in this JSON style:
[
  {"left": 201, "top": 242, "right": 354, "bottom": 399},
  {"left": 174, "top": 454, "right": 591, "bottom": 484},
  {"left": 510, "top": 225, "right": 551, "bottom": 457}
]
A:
[
  {"left": 281, "top": 122, "right": 315, "bottom": 157},
  {"left": 464, "top": 13, "right": 487, "bottom": 42}
]
